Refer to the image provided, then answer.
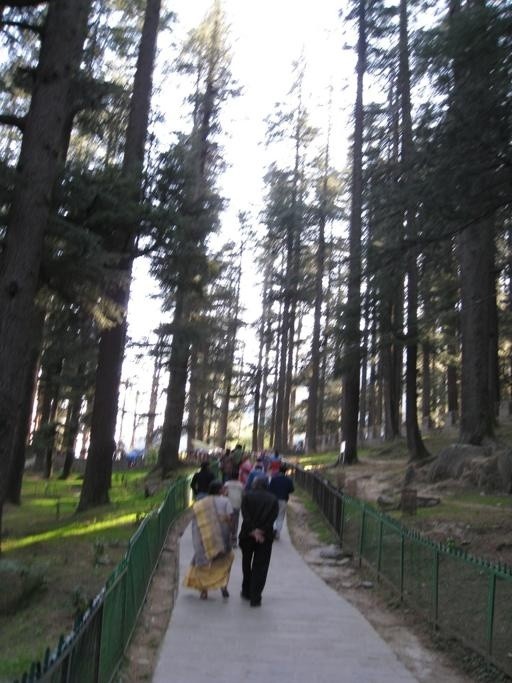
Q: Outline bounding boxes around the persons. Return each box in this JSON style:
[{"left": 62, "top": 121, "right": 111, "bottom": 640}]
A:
[
  {"left": 184, "top": 480, "right": 236, "bottom": 600},
  {"left": 190, "top": 444, "right": 294, "bottom": 548},
  {"left": 238, "top": 474, "right": 279, "bottom": 606}
]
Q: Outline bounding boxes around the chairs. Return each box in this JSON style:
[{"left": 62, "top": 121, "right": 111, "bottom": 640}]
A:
[
  {"left": 200, "top": 590, "right": 208, "bottom": 600},
  {"left": 240, "top": 590, "right": 262, "bottom": 607},
  {"left": 220, "top": 588, "right": 229, "bottom": 598}
]
